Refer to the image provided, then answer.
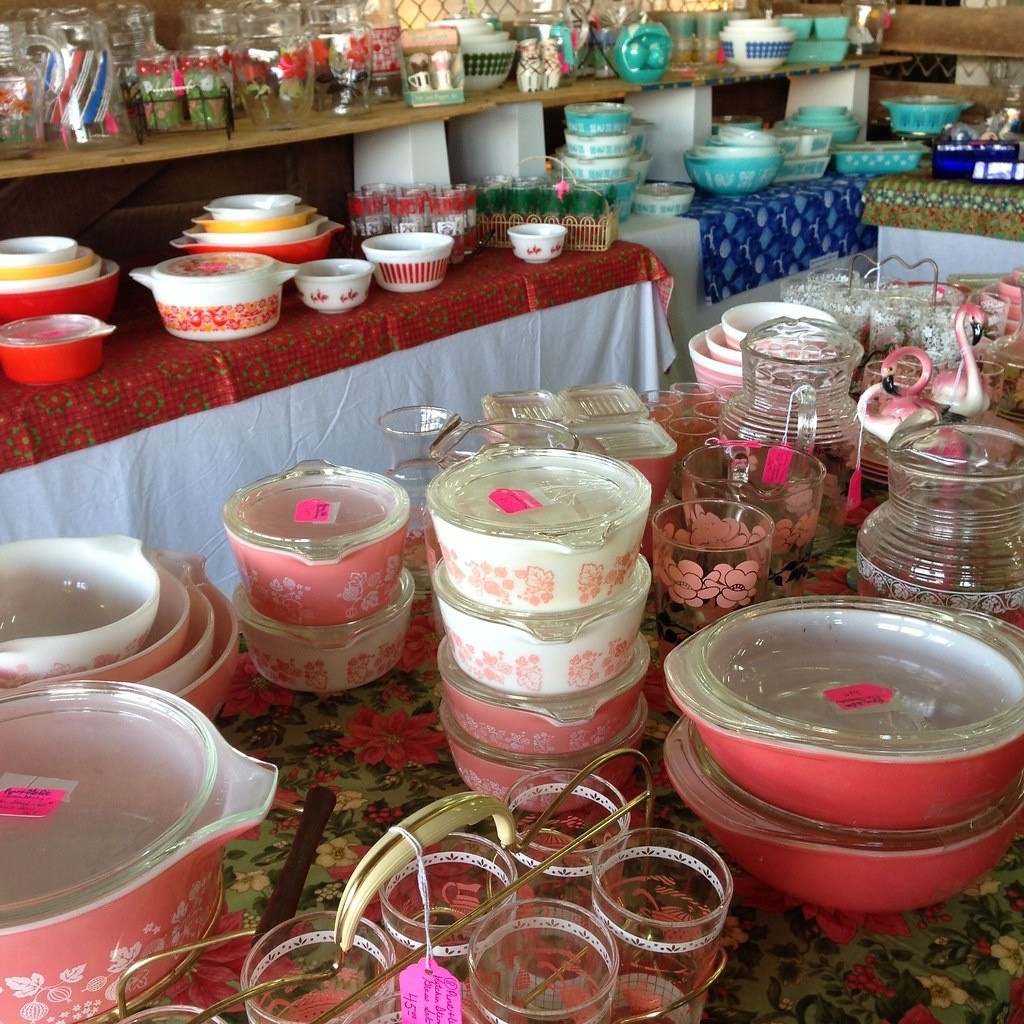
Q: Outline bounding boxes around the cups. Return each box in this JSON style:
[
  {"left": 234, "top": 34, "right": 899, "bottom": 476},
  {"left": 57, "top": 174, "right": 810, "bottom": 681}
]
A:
[
  {"left": 779, "top": 265, "right": 1024, "bottom": 488},
  {"left": 591, "top": 826, "right": 733, "bottom": 1024},
  {"left": 115, "top": 1006, "right": 226, "bottom": 1024},
  {"left": 342, "top": 991, "right": 483, "bottom": 1024},
  {"left": 468, "top": 899, "right": 621, "bottom": 1024},
  {"left": 240, "top": 911, "right": 396, "bottom": 1024},
  {"left": 378, "top": 830, "right": 517, "bottom": 992},
  {"left": 681, "top": 441, "right": 828, "bottom": 601},
  {"left": 651, "top": 499, "right": 777, "bottom": 717},
  {"left": 642, "top": 381, "right": 746, "bottom": 461},
  {"left": 503, "top": 766, "right": 630, "bottom": 1010}
]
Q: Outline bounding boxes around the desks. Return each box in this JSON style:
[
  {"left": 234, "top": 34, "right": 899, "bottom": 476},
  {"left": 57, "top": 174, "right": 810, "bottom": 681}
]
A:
[{"left": 0, "top": 0, "right": 1023, "bottom": 1022}]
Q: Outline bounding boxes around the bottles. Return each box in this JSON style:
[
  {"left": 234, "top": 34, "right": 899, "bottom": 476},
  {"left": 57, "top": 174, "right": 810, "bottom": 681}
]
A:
[
  {"left": 856, "top": 423, "right": 1022, "bottom": 633},
  {"left": 376, "top": 407, "right": 460, "bottom": 593},
  {"left": 719, "top": 314, "right": 864, "bottom": 562}
]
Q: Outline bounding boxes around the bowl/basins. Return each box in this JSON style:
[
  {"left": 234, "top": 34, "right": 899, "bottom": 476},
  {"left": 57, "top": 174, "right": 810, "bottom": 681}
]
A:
[
  {"left": 425, "top": 441, "right": 651, "bottom": 613},
  {"left": 432, "top": 550, "right": 651, "bottom": 696},
  {"left": 115, "top": 547, "right": 216, "bottom": 696},
  {"left": 1, "top": 0, "right": 1023, "bottom": 385},
  {"left": 178, "top": 552, "right": 240, "bottom": 720},
  {"left": 1, "top": 533, "right": 162, "bottom": 691},
  {"left": 664, "top": 711, "right": 1024, "bottom": 911},
  {"left": 437, "top": 631, "right": 651, "bottom": 755},
  {"left": 480, "top": 383, "right": 678, "bottom": 560},
  {"left": 0, "top": 551, "right": 191, "bottom": 685},
  {"left": 664, "top": 594, "right": 1022, "bottom": 832},
  {"left": 232, "top": 567, "right": 415, "bottom": 693},
  {"left": 1, "top": 680, "right": 279, "bottom": 1024},
  {"left": 222, "top": 459, "right": 410, "bottom": 625},
  {"left": 440, "top": 690, "right": 648, "bottom": 814}
]
